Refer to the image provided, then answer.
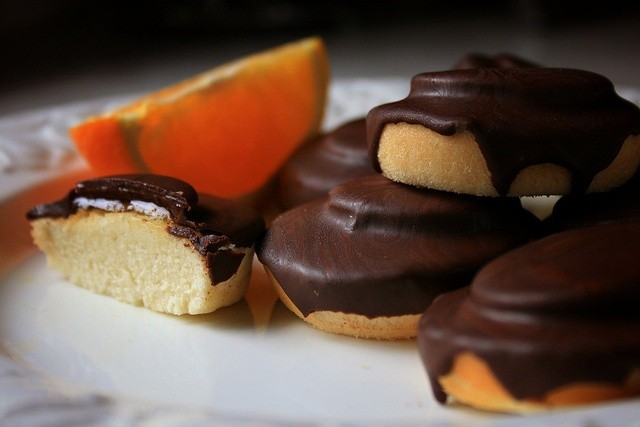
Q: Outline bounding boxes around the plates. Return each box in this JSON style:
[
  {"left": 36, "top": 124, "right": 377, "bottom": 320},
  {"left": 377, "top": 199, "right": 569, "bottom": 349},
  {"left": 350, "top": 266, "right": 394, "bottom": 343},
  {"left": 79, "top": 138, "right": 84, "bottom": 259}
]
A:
[{"left": 0, "top": 75, "right": 640, "bottom": 427}]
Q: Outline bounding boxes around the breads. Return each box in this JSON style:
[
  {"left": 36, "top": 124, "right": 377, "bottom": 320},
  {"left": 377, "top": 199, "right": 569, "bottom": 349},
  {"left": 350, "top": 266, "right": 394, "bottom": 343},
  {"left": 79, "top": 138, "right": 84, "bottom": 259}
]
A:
[
  {"left": 268, "top": 120, "right": 368, "bottom": 214},
  {"left": 418, "top": 217, "right": 639, "bottom": 416},
  {"left": 367, "top": 54, "right": 640, "bottom": 198},
  {"left": 26, "top": 174, "right": 255, "bottom": 318},
  {"left": 255, "top": 178, "right": 526, "bottom": 341}
]
